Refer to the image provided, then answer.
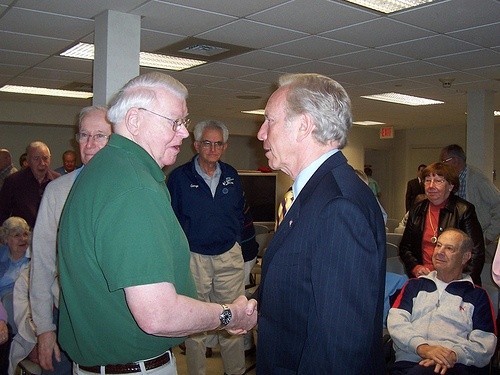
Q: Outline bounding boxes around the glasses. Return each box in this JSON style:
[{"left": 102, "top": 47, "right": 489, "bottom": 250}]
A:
[
  {"left": 440, "top": 158, "right": 453, "bottom": 164},
  {"left": 75, "top": 132, "right": 110, "bottom": 143},
  {"left": 138, "top": 106, "right": 191, "bottom": 132},
  {"left": 422, "top": 177, "right": 448, "bottom": 184},
  {"left": 198, "top": 140, "right": 224, "bottom": 147}
]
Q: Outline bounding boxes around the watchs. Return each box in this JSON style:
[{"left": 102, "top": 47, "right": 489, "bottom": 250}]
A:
[{"left": 215, "top": 303, "right": 232, "bottom": 331}]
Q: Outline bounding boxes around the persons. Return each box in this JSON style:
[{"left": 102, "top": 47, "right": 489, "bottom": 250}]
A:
[
  {"left": 399, "top": 162, "right": 486, "bottom": 287},
  {"left": 241, "top": 189, "right": 260, "bottom": 285},
  {"left": 364, "top": 168, "right": 382, "bottom": 198},
  {"left": 440, "top": 144, "right": 500, "bottom": 245},
  {"left": 166, "top": 118, "right": 245, "bottom": 375},
  {"left": 0, "top": 141, "right": 81, "bottom": 375},
  {"left": 406, "top": 164, "right": 427, "bottom": 213},
  {"left": 31, "top": 105, "right": 113, "bottom": 375},
  {"left": 57, "top": 71, "right": 258, "bottom": 375},
  {"left": 386, "top": 227, "right": 498, "bottom": 375},
  {"left": 230, "top": 73, "right": 387, "bottom": 375}
]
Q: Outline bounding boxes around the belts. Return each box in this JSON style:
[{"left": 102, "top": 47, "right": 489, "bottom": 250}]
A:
[{"left": 79, "top": 353, "right": 172, "bottom": 374}]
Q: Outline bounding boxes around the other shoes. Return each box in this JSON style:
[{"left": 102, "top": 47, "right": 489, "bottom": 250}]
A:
[{"left": 205, "top": 347, "right": 213, "bottom": 357}]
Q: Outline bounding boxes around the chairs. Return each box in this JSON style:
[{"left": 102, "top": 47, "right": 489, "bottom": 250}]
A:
[{"left": 178, "top": 224, "right": 500, "bottom": 375}]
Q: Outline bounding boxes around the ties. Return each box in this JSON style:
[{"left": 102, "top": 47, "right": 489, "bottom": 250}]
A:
[{"left": 273, "top": 185, "right": 296, "bottom": 232}]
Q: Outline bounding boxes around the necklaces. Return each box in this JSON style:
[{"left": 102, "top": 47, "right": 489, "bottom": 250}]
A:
[{"left": 428, "top": 204, "right": 438, "bottom": 243}]
